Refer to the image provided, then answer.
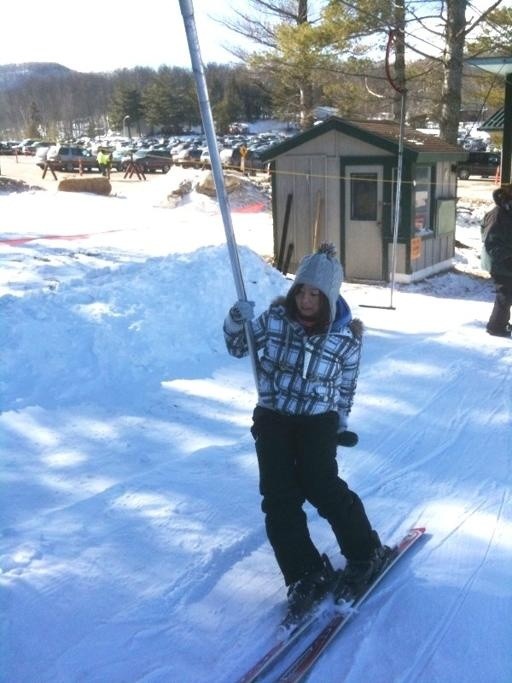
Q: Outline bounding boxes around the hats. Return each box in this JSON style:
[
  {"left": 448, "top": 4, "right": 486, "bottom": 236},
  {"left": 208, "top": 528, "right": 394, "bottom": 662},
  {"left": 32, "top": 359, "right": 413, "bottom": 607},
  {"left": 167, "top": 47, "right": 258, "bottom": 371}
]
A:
[{"left": 293, "top": 243, "right": 344, "bottom": 323}]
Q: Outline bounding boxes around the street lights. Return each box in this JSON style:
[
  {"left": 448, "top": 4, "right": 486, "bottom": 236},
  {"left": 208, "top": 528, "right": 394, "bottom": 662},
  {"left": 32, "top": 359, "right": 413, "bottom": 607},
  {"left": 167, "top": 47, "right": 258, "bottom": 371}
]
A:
[{"left": 123, "top": 115, "right": 130, "bottom": 137}]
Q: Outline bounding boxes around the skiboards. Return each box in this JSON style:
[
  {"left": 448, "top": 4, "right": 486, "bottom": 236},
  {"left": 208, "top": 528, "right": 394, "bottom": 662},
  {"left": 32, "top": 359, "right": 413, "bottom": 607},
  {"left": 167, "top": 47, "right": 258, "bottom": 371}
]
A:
[{"left": 235, "top": 528, "right": 425, "bottom": 681}]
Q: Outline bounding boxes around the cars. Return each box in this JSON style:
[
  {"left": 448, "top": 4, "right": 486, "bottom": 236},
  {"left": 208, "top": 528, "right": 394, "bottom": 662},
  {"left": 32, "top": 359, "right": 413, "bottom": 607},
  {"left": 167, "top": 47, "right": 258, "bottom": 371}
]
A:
[{"left": 0, "top": 123, "right": 281, "bottom": 174}]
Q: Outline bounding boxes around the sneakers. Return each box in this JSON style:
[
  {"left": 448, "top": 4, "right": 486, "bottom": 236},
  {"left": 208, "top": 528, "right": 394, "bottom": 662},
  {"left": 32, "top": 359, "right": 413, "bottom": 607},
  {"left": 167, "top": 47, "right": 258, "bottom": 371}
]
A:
[{"left": 278, "top": 529, "right": 393, "bottom": 643}]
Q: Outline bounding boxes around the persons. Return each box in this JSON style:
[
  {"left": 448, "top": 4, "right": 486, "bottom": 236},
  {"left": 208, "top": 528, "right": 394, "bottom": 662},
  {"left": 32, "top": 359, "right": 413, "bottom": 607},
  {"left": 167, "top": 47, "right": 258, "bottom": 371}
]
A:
[
  {"left": 478, "top": 187, "right": 511, "bottom": 336},
  {"left": 222, "top": 240, "right": 396, "bottom": 629}
]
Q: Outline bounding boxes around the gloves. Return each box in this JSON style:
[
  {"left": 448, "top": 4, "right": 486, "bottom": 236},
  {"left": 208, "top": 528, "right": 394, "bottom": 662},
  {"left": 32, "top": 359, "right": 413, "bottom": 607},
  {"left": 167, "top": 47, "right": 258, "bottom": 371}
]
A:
[
  {"left": 229, "top": 300, "right": 255, "bottom": 325},
  {"left": 336, "top": 412, "right": 350, "bottom": 435}
]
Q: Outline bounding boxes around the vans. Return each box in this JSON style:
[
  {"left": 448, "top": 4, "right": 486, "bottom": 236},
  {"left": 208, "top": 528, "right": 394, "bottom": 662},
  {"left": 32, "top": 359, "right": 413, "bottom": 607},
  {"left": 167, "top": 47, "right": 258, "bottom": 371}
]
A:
[{"left": 451, "top": 152, "right": 501, "bottom": 180}]
